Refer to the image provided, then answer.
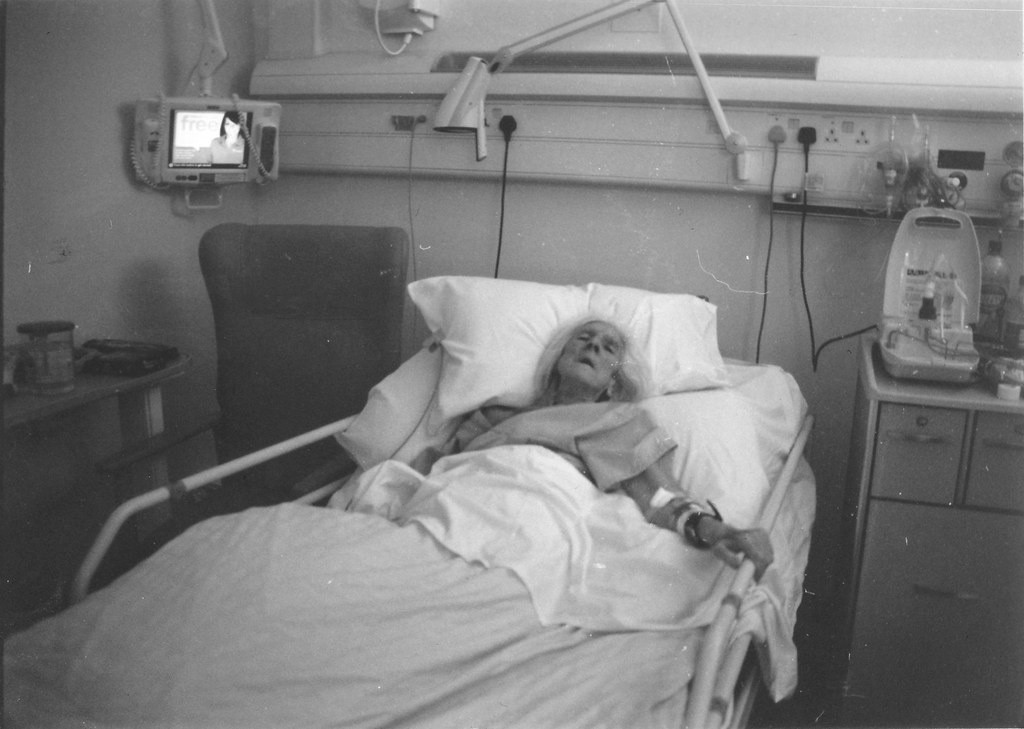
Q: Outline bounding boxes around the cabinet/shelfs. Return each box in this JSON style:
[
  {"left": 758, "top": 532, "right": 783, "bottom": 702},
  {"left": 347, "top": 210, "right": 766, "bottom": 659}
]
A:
[
  {"left": 0, "top": 351, "right": 192, "bottom": 565},
  {"left": 833, "top": 335, "right": 1024, "bottom": 711}
]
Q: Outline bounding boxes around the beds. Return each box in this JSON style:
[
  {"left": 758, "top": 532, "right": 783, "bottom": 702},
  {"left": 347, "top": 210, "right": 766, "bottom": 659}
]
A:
[{"left": 0, "top": 357, "right": 817, "bottom": 729}]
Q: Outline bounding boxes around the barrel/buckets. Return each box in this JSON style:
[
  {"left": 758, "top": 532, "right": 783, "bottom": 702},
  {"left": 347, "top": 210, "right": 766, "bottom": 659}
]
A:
[{"left": 17, "top": 318, "right": 79, "bottom": 387}]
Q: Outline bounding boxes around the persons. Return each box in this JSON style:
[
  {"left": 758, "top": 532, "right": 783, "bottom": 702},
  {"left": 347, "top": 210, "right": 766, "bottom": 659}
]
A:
[
  {"left": 407, "top": 316, "right": 775, "bottom": 583},
  {"left": 210, "top": 111, "right": 246, "bottom": 163}
]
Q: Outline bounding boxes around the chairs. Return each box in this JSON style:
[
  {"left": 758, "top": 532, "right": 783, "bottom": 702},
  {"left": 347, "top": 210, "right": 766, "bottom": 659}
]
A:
[{"left": 94, "top": 225, "right": 410, "bottom": 559}]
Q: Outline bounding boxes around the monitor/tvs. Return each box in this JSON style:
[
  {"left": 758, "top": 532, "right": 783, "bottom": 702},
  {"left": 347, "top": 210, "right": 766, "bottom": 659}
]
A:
[{"left": 168, "top": 108, "right": 253, "bottom": 169}]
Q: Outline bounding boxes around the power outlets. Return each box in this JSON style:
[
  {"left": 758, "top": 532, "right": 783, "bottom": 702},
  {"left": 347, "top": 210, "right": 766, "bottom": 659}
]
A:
[{"left": 771, "top": 114, "right": 888, "bottom": 153}]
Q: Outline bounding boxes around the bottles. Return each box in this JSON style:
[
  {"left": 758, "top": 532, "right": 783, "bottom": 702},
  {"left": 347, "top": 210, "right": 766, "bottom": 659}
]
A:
[{"left": 981, "top": 241, "right": 1011, "bottom": 346}]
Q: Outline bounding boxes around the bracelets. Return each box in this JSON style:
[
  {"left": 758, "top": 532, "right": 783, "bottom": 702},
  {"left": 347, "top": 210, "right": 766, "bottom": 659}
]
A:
[{"left": 685, "top": 499, "right": 724, "bottom": 547}]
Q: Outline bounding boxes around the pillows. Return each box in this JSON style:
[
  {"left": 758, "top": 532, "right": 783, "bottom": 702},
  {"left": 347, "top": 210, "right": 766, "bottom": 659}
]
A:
[{"left": 406, "top": 276, "right": 730, "bottom": 437}]
